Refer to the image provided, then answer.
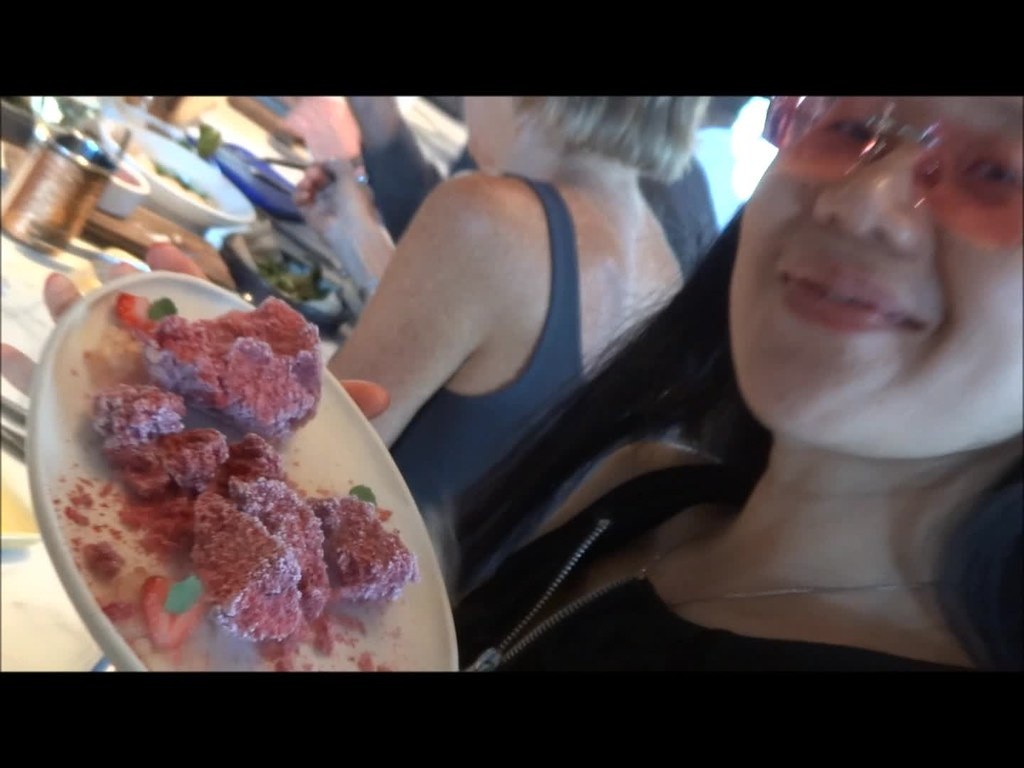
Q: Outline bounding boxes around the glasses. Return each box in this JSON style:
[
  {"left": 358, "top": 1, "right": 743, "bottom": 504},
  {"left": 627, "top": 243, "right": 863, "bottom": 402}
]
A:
[{"left": 762, "top": 97, "right": 1024, "bottom": 248}]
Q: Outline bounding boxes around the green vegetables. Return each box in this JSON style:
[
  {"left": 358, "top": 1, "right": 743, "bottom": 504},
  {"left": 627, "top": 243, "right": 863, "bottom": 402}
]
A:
[
  {"left": 349, "top": 485, "right": 377, "bottom": 506},
  {"left": 198, "top": 122, "right": 223, "bottom": 159},
  {"left": 255, "top": 254, "right": 328, "bottom": 300},
  {"left": 164, "top": 572, "right": 203, "bottom": 614},
  {"left": 149, "top": 297, "right": 177, "bottom": 320}
]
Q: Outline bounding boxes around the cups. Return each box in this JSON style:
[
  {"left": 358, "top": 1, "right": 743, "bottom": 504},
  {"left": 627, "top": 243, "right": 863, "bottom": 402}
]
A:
[{"left": 1, "top": 125, "right": 115, "bottom": 255}]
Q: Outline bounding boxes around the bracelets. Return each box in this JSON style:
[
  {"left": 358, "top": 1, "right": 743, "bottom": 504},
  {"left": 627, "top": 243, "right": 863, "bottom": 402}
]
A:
[{"left": 320, "top": 146, "right": 376, "bottom": 188}]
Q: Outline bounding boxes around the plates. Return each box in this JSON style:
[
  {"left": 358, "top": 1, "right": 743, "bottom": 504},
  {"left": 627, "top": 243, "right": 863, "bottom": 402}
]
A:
[
  {"left": 29, "top": 96, "right": 358, "bottom": 344},
  {"left": 25, "top": 268, "right": 459, "bottom": 671}
]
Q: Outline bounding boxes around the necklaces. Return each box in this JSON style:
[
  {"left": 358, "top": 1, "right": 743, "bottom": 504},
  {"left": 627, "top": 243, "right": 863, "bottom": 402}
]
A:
[{"left": 635, "top": 518, "right": 947, "bottom": 610}]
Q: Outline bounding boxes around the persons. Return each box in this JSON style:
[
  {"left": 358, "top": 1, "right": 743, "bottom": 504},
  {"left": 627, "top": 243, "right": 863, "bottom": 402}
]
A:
[
  {"left": 1, "top": 97, "right": 1024, "bottom": 673},
  {"left": 196, "top": 96, "right": 755, "bottom": 528}
]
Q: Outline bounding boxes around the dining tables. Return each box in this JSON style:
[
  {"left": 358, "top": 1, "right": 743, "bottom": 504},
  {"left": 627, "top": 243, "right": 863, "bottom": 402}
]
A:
[{"left": 0, "top": 95, "right": 468, "bottom": 674}]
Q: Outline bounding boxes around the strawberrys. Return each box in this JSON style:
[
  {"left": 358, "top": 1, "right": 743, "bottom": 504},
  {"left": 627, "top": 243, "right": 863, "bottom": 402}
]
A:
[
  {"left": 143, "top": 576, "right": 206, "bottom": 651},
  {"left": 117, "top": 291, "right": 157, "bottom": 330}
]
